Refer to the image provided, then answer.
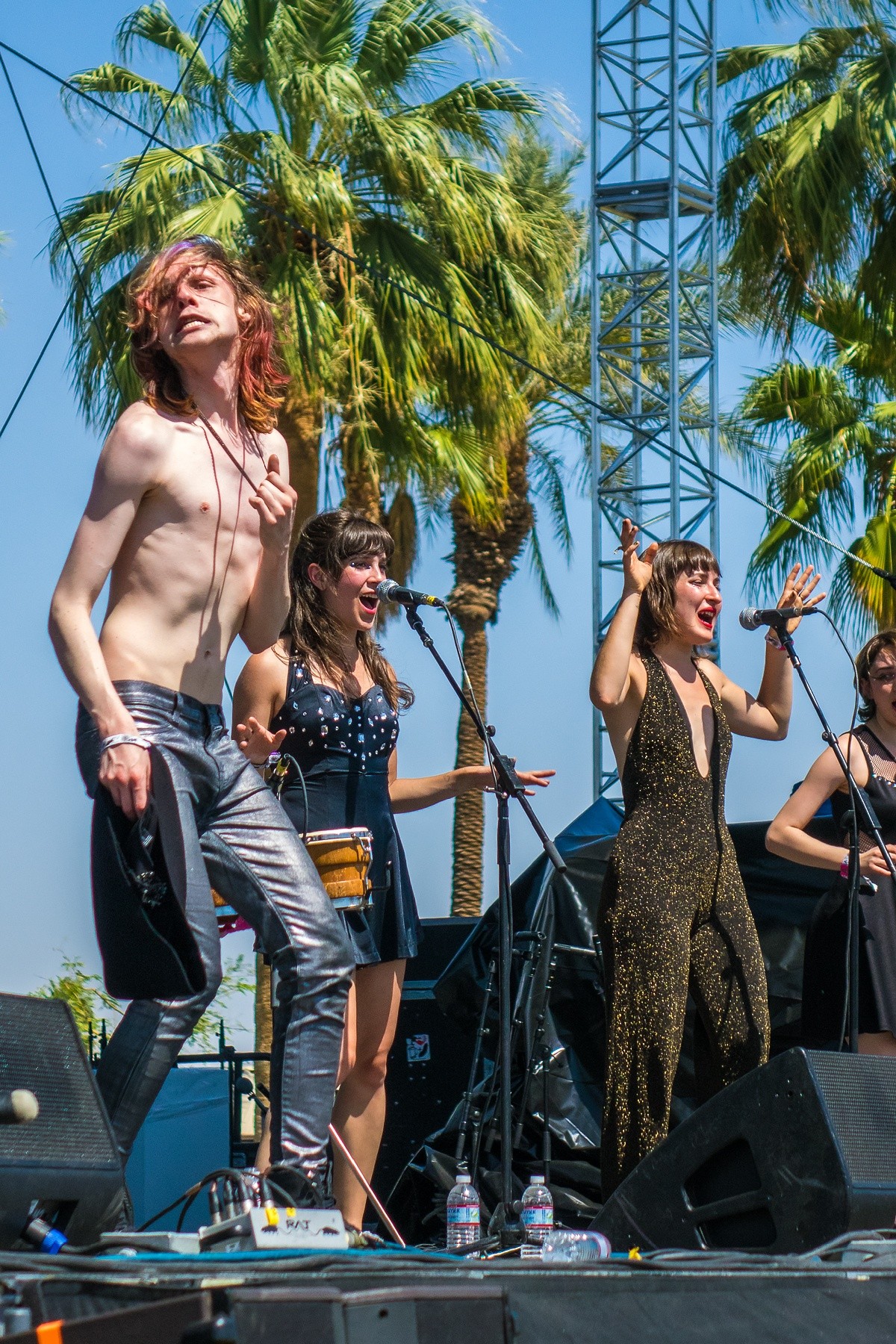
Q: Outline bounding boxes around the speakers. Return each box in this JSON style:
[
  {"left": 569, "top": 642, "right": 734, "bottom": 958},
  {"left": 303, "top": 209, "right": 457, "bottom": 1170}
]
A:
[
  {"left": 587, "top": 1046, "right": 895, "bottom": 1257},
  {"left": 0, "top": 991, "right": 125, "bottom": 1253}
]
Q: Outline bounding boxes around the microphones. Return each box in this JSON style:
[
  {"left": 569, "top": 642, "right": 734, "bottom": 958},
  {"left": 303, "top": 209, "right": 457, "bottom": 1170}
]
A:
[
  {"left": 0, "top": 1087, "right": 39, "bottom": 1126},
  {"left": 739, "top": 605, "right": 817, "bottom": 631},
  {"left": 377, "top": 578, "right": 444, "bottom": 607},
  {"left": 265, "top": 753, "right": 292, "bottom": 798}
]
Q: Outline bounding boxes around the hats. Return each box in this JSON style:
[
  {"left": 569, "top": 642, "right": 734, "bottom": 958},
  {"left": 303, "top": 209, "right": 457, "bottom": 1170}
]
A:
[{"left": 90, "top": 781, "right": 207, "bottom": 1001}]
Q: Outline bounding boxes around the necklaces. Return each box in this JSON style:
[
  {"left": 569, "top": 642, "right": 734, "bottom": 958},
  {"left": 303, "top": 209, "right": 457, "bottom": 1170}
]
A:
[{"left": 179, "top": 389, "right": 269, "bottom": 494}]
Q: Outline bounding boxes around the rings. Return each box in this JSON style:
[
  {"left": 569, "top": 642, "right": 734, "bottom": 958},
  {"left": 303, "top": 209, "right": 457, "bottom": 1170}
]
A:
[
  {"left": 800, "top": 594, "right": 804, "bottom": 600},
  {"left": 794, "top": 588, "right": 799, "bottom": 593}
]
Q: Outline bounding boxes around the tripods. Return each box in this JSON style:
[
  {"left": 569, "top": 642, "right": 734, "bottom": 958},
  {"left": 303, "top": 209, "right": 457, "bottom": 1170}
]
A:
[{"left": 404, "top": 610, "right": 567, "bottom": 1259}]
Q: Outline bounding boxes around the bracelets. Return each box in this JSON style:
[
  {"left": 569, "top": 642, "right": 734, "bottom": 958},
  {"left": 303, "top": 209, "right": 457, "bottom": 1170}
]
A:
[
  {"left": 764, "top": 634, "right": 794, "bottom": 651},
  {"left": 95, "top": 731, "right": 154, "bottom": 755},
  {"left": 841, "top": 854, "right": 850, "bottom": 879},
  {"left": 250, "top": 758, "right": 271, "bottom": 770}
]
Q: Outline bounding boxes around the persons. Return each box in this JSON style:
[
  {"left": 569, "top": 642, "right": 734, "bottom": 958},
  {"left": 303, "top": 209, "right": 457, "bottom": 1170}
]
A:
[
  {"left": 231, "top": 510, "right": 556, "bottom": 1253},
  {"left": 765, "top": 632, "right": 896, "bottom": 1057},
  {"left": 588, "top": 517, "right": 829, "bottom": 1204},
  {"left": 49, "top": 242, "right": 384, "bottom": 1270}
]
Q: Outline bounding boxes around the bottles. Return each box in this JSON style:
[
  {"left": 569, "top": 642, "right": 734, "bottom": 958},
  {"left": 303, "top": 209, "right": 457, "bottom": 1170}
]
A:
[
  {"left": 541, "top": 1230, "right": 611, "bottom": 1262},
  {"left": 446, "top": 1175, "right": 480, "bottom": 1258},
  {"left": 520, "top": 1176, "right": 554, "bottom": 1260}
]
